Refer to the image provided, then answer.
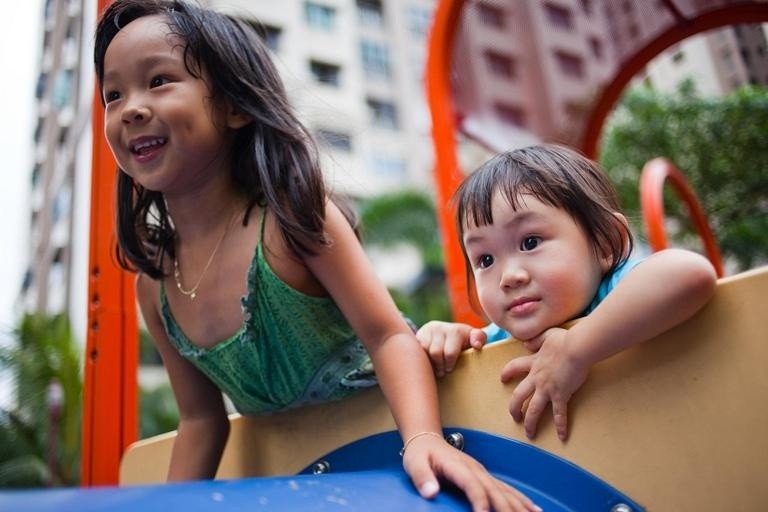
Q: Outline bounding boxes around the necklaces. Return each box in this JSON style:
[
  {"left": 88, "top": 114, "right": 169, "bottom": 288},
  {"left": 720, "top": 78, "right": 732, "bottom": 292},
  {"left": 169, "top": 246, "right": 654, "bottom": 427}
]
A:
[{"left": 174, "top": 218, "right": 233, "bottom": 300}]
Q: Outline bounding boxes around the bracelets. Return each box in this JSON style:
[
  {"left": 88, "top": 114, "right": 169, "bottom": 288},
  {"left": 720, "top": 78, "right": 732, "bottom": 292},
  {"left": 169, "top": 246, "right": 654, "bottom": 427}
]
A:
[{"left": 399, "top": 432, "right": 445, "bottom": 457}]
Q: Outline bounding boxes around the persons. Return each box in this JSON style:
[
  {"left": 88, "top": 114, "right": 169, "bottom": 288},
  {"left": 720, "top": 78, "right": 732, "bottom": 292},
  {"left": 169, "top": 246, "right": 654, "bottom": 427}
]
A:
[
  {"left": 93, "top": 0, "right": 547, "bottom": 512},
  {"left": 415, "top": 143, "right": 717, "bottom": 443}
]
